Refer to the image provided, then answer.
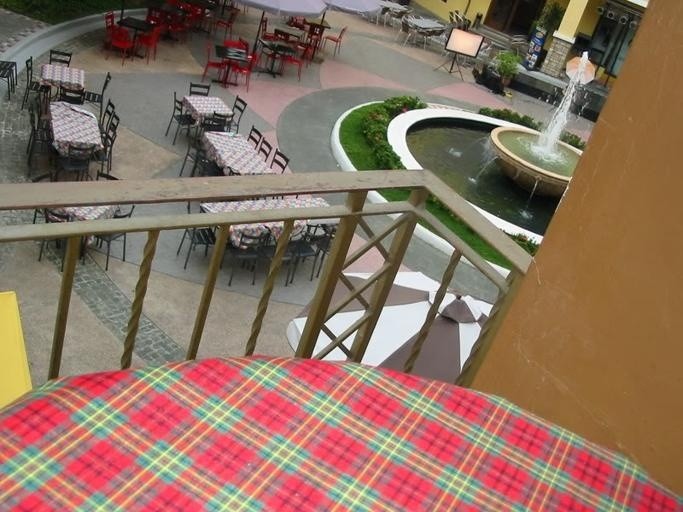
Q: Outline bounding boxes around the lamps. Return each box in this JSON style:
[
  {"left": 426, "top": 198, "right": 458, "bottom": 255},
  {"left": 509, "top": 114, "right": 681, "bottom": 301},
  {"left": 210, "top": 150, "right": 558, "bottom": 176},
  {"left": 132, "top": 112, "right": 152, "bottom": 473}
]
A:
[{"left": 380, "top": 2, "right": 446, "bottom": 52}]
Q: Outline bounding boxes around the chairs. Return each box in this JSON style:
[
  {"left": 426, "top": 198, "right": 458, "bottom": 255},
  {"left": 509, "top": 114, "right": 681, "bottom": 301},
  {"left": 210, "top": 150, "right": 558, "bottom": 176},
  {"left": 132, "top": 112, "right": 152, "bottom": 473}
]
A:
[
  {"left": 380, "top": 2, "right": 446, "bottom": 52},
  {"left": 179, "top": 117, "right": 291, "bottom": 178},
  {"left": 2, "top": 61, "right": 18, "bottom": 101},
  {"left": 105, "top": 1, "right": 342, "bottom": 91},
  {"left": 176, "top": 194, "right": 342, "bottom": 286},
  {"left": 21, "top": 48, "right": 136, "bottom": 272},
  {"left": 165, "top": 81, "right": 248, "bottom": 145}
]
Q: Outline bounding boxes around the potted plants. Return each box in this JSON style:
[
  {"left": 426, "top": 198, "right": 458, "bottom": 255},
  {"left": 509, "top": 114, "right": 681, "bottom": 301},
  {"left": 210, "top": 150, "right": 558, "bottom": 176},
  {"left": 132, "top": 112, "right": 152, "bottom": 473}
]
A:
[{"left": 494, "top": 48, "right": 523, "bottom": 88}]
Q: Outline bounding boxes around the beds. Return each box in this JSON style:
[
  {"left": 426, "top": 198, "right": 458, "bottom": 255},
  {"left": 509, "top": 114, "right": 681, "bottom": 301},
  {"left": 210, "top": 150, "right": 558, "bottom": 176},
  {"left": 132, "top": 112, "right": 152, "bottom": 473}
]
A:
[
  {"left": 320, "top": 1, "right": 381, "bottom": 24},
  {"left": 234, "top": 1, "right": 328, "bottom": 54}
]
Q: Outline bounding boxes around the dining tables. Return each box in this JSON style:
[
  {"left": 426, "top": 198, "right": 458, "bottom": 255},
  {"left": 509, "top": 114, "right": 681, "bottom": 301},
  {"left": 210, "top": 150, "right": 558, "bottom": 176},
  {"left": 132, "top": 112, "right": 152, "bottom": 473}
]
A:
[{"left": 494, "top": 48, "right": 523, "bottom": 88}]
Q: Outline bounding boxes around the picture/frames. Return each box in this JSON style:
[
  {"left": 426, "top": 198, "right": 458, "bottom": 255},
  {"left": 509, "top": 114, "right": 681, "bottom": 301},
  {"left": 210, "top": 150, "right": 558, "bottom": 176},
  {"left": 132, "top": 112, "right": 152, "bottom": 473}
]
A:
[{"left": 1, "top": 354, "right": 679, "bottom": 511}]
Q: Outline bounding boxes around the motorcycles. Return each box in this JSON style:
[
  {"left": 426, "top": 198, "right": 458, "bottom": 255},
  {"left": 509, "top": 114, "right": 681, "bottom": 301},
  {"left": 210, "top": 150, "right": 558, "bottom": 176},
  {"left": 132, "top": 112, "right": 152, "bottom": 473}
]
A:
[{"left": 433, "top": 53, "right": 465, "bottom": 82}]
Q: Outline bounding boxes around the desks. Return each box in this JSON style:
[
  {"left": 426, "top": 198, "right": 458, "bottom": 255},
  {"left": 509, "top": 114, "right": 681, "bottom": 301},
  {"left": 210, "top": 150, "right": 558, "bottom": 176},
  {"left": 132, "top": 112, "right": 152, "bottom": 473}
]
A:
[{"left": 1, "top": 354, "right": 679, "bottom": 511}]
